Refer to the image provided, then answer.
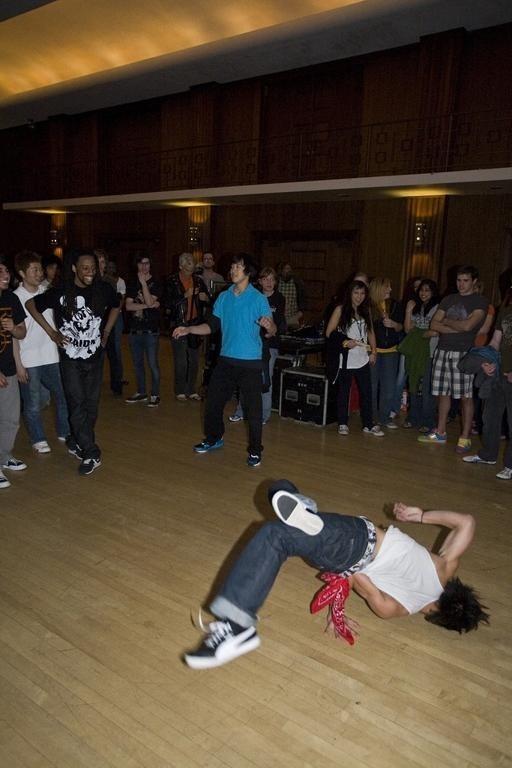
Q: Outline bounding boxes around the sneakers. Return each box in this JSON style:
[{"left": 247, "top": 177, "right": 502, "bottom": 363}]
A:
[
  {"left": 364, "top": 425, "right": 384, "bottom": 437},
  {"left": 417, "top": 433, "right": 447, "bottom": 444},
  {"left": 125, "top": 393, "right": 148, "bottom": 404},
  {"left": 183, "top": 621, "right": 261, "bottom": 671},
  {"left": 1, "top": 458, "right": 27, "bottom": 472},
  {"left": 456, "top": 437, "right": 472, "bottom": 454},
  {"left": 462, "top": 453, "right": 496, "bottom": 465},
  {"left": 31, "top": 440, "right": 51, "bottom": 453},
  {"left": 339, "top": 424, "right": 350, "bottom": 435},
  {"left": 386, "top": 422, "right": 397, "bottom": 429},
  {"left": 0, "top": 474, "right": 11, "bottom": 488},
  {"left": 270, "top": 490, "right": 323, "bottom": 537},
  {"left": 247, "top": 454, "right": 262, "bottom": 467},
  {"left": 59, "top": 434, "right": 101, "bottom": 475},
  {"left": 175, "top": 393, "right": 203, "bottom": 400},
  {"left": 149, "top": 395, "right": 159, "bottom": 407},
  {"left": 496, "top": 468, "right": 512, "bottom": 479},
  {"left": 229, "top": 414, "right": 247, "bottom": 421},
  {"left": 193, "top": 437, "right": 224, "bottom": 452}
]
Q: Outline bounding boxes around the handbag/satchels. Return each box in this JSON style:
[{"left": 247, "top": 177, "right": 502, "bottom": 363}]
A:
[{"left": 188, "top": 319, "right": 201, "bottom": 349}]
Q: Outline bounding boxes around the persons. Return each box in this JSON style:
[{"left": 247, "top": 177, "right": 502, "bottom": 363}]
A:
[
  {"left": 172, "top": 252, "right": 307, "bottom": 466},
  {"left": 0, "top": 250, "right": 127, "bottom": 488},
  {"left": 124, "top": 251, "right": 226, "bottom": 408},
  {"left": 322, "top": 265, "right": 512, "bottom": 480},
  {"left": 184, "top": 479, "right": 491, "bottom": 670}
]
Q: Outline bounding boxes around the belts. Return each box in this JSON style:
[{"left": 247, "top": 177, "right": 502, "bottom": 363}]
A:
[{"left": 130, "top": 329, "right": 157, "bottom": 335}]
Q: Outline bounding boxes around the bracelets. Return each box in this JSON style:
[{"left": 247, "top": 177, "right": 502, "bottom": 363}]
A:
[{"left": 421, "top": 510, "right": 424, "bottom": 524}]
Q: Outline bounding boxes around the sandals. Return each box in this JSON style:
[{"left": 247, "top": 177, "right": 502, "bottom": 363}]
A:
[{"left": 401, "top": 420, "right": 431, "bottom": 432}]
